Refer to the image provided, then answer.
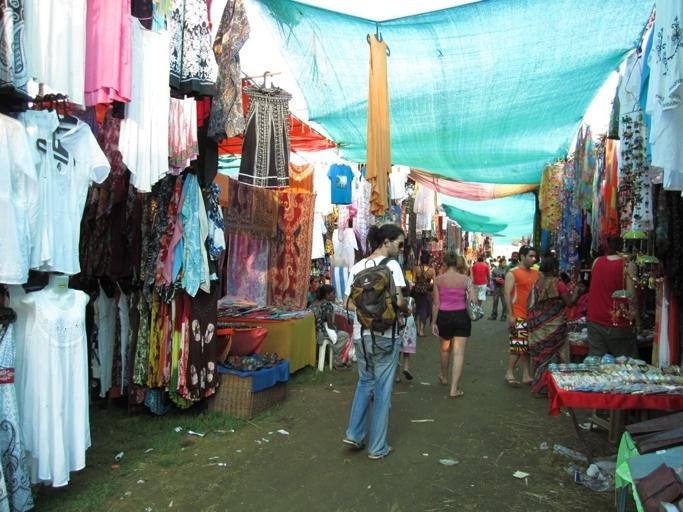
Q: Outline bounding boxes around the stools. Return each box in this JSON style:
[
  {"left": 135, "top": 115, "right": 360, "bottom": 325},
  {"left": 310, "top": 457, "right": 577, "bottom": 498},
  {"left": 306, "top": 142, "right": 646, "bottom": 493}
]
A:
[{"left": 314, "top": 337, "right": 332, "bottom": 371}]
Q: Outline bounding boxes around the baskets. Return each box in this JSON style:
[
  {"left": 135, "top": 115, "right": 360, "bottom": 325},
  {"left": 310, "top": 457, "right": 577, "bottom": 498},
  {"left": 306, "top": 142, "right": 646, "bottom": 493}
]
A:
[{"left": 207, "top": 360, "right": 289, "bottom": 422}]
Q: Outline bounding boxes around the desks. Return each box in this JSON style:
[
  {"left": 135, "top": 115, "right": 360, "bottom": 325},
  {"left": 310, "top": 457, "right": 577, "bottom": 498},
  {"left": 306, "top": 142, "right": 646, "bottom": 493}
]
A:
[
  {"left": 530, "top": 358, "right": 682, "bottom": 447},
  {"left": 214, "top": 307, "right": 316, "bottom": 373}
]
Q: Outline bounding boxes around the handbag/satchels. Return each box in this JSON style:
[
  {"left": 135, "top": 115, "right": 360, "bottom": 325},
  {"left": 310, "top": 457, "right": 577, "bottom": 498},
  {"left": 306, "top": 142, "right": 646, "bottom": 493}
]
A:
[{"left": 465, "top": 278, "right": 485, "bottom": 321}]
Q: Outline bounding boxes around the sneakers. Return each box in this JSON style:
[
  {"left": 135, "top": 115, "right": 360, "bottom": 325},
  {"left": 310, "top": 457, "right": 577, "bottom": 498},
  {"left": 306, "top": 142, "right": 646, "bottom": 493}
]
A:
[
  {"left": 343, "top": 439, "right": 391, "bottom": 460},
  {"left": 488, "top": 314, "right": 506, "bottom": 320}
]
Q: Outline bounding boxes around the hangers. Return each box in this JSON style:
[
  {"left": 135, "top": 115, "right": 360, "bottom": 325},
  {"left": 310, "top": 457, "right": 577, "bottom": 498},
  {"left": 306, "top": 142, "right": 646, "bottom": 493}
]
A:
[
  {"left": 35, "top": 128, "right": 77, "bottom": 169},
  {"left": 48, "top": 93, "right": 82, "bottom": 135},
  {"left": 365, "top": 19, "right": 392, "bottom": 58},
  {"left": 258, "top": 70, "right": 281, "bottom": 95}
]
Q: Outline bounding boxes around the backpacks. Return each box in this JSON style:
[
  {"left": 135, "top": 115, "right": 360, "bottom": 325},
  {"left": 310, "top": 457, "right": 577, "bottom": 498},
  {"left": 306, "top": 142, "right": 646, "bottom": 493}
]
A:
[
  {"left": 349, "top": 257, "right": 406, "bottom": 336},
  {"left": 414, "top": 266, "right": 428, "bottom": 294}
]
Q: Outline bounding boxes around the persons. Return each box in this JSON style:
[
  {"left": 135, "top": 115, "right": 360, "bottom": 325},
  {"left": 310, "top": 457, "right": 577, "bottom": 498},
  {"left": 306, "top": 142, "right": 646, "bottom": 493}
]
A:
[
  {"left": 502, "top": 242, "right": 544, "bottom": 388},
  {"left": 342, "top": 223, "right": 414, "bottom": 461},
  {"left": 427, "top": 251, "right": 482, "bottom": 399},
  {"left": 411, "top": 234, "right": 536, "bottom": 339},
  {"left": 585, "top": 231, "right": 645, "bottom": 362},
  {"left": 306, "top": 282, "right": 349, "bottom": 373},
  {"left": 396, "top": 279, "right": 418, "bottom": 382},
  {"left": 307, "top": 272, "right": 319, "bottom": 307},
  {"left": 522, "top": 254, "right": 581, "bottom": 397}
]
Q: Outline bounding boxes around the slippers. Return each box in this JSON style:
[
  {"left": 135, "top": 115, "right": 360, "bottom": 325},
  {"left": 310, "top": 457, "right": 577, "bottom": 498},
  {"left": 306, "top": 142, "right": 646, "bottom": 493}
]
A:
[
  {"left": 505, "top": 376, "right": 535, "bottom": 387},
  {"left": 438, "top": 373, "right": 464, "bottom": 399}
]
